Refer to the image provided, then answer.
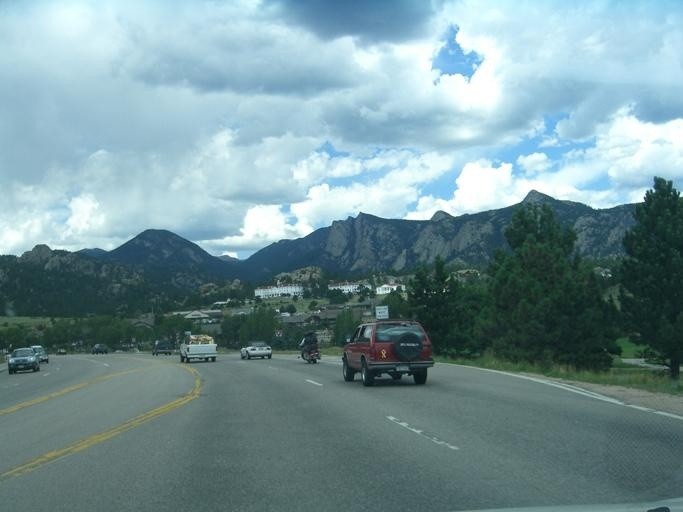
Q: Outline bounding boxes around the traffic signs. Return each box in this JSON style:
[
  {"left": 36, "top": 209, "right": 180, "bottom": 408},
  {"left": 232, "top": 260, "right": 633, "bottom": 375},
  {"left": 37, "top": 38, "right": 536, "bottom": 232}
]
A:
[{"left": 375, "top": 305, "right": 389, "bottom": 320}]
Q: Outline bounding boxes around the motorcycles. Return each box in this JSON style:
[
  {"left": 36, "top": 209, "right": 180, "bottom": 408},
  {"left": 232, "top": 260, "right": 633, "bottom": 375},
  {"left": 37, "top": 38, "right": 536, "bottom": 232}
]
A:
[{"left": 296, "top": 341, "right": 319, "bottom": 364}]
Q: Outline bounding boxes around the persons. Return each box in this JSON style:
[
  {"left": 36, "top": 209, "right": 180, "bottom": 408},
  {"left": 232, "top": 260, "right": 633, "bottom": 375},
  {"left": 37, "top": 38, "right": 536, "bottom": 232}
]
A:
[{"left": 299, "top": 329, "right": 318, "bottom": 362}]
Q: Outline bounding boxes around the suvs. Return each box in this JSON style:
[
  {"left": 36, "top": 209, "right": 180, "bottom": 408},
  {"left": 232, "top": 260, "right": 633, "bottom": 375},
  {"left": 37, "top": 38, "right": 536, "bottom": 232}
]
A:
[{"left": 341, "top": 318, "right": 435, "bottom": 386}]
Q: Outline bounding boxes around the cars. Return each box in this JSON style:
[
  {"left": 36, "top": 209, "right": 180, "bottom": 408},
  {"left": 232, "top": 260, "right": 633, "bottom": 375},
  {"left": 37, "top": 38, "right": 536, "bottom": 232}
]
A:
[
  {"left": 111, "top": 341, "right": 134, "bottom": 353},
  {"left": 172, "top": 303, "right": 351, "bottom": 325},
  {"left": 8, "top": 347, "right": 40, "bottom": 376},
  {"left": 55, "top": 349, "right": 68, "bottom": 356},
  {"left": 91, "top": 343, "right": 108, "bottom": 356},
  {"left": 151, "top": 340, "right": 174, "bottom": 356},
  {"left": 30, "top": 345, "right": 50, "bottom": 365},
  {"left": 238, "top": 341, "right": 271, "bottom": 360}
]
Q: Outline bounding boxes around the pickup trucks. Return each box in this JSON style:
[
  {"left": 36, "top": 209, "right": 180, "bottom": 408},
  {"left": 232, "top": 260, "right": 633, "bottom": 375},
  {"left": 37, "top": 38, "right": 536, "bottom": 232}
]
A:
[{"left": 178, "top": 335, "right": 217, "bottom": 363}]
